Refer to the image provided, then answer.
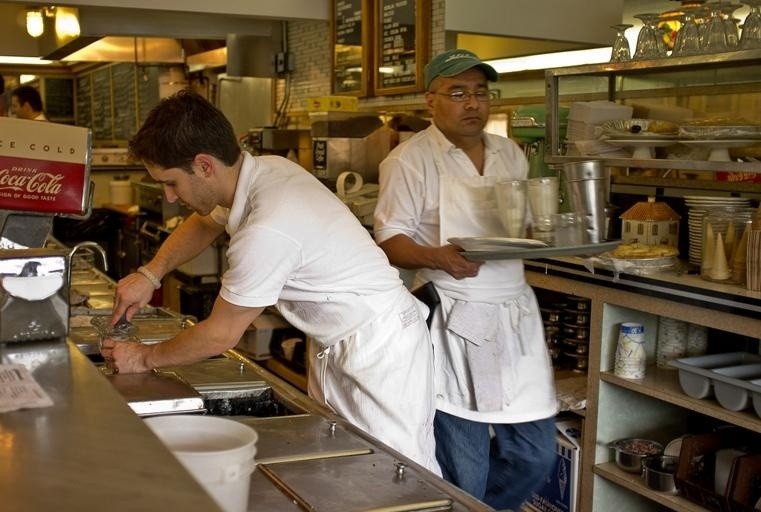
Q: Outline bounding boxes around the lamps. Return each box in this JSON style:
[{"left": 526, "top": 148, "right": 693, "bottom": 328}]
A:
[
  {"left": 56, "top": 6, "right": 81, "bottom": 43},
  {"left": 26, "top": 6, "right": 44, "bottom": 38}
]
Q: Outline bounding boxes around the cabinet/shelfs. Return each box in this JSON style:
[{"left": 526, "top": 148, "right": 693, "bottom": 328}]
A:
[
  {"left": 544, "top": 50, "right": 761, "bottom": 201},
  {"left": 588, "top": 301, "right": 761, "bottom": 512}
]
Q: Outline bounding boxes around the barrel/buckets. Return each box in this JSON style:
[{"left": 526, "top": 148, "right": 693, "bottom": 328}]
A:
[{"left": 141, "top": 413, "right": 258, "bottom": 511}]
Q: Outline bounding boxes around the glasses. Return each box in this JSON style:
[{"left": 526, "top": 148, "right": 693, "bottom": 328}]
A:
[{"left": 430, "top": 90, "right": 490, "bottom": 102}]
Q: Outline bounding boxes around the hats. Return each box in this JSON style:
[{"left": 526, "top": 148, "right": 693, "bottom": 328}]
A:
[{"left": 422, "top": 48, "right": 498, "bottom": 90}]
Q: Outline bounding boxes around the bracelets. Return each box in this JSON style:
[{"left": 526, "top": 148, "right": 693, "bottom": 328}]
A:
[{"left": 138, "top": 267, "right": 161, "bottom": 290}]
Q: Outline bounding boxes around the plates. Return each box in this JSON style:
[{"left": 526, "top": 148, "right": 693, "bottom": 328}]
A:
[
  {"left": 683, "top": 194, "right": 749, "bottom": 269},
  {"left": 604, "top": 139, "right": 679, "bottom": 160},
  {"left": 447, "top": 237, "right": 547, "bottom": 252},
  {"left": 664, "top": 436, "right": 685, "bottom": 470},
  {"left": 616, "top": 257, "right": 675, "bottom": 274},
  {"left": 462, "top": 239, "right": 623, "bottom": 262},
  {"left": 678, "top": 139, "right": 760, "bottom": 162}
]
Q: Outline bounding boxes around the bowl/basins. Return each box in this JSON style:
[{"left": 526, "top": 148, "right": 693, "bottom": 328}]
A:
[
  {"left": 609, "top": 438, "right": 663, "bottom": 474},
  {"left": 642, "top": 455, "right": 686, "bottom": 494}
]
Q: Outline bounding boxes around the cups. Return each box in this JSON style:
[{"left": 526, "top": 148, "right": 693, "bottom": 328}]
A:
[
  {"left": 703, "top": 1, "right": 731, "bottom": 52},
  {"left": 676, "top": 6, "right": 701, "bottom": 56},
  {"left": 653, "top": 17, "right": 667, "bottom": 58},
  {"left": 702, "top": 16, "right": 729, "bottom": 47},
  {"left": 657, "top": 320, "right": 686, "bottom": 370},
  {"left": 531, "top": 216, "right": 553, "bottom": 243},
  {"left": 608, "top": 24, "right": 632, "bottom": 62},
  {"left": 730, "top": 19, "right": 741, "bottom": 41},
  {"left": 615, "top": 323, "right": 647, "bottom": 379},
  {"left": 670, "top": 15, "right": 686, "bottom": 58},
  {"left": 495, "top": 180, "right": 527, "bottom": 237},
  {"left": 722, "top": 5, "right": 744, "bottom": 51},
  {"left": 632, "top": 13, "right": 662, "bottom": 61},
  {"left": 736, "top": 0, "right": 761, "bottom": 51},
  {"left": 687, "top": 324, "right": 708, "bottom": 354},
  {"left": 527, "top": 177, "right": 561, "bottom": 230},
  {"left": 553, "top": 211, "right": 584, "bottom": 247}
]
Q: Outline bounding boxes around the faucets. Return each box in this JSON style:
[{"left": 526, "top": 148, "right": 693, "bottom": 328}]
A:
[{"left": 65, "top": 240, "right": 110, "bottom": 289}]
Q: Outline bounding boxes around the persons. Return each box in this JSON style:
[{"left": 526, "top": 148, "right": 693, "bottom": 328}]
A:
[
  {"left": 373, "top": 49, "right": 605, "bottom": 510},
  {"left": 100, "top": 91, "right": 443, "bottom": 479},
  {"left": 10, "top": 85, "right": 48, "bottom": 121}
]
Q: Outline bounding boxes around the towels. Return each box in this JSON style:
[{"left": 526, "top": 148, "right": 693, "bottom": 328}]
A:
[{"left": 444, "top": 297, "right": 506, "bottom": 415}]
